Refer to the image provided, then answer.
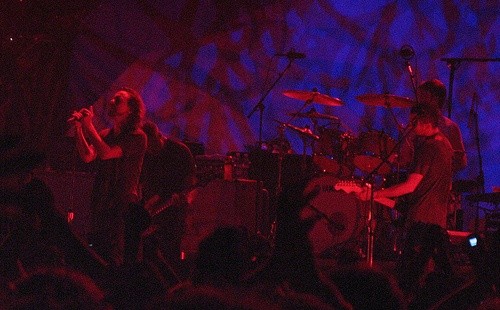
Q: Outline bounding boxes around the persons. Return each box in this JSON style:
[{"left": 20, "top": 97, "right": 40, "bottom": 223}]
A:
[
  {"left": 73, "top": 88, "right": 148, "bottom": 229},
  {"left": 417, "top": 79, "right": 468, "bottom": 174},
  {"left": 0, "top": 178, "right": 500, "bottom": 310},
  {"left": 357, "top": 100, "right": 454, "bottom": 293},
  {"left": 140, "top": 121, "right": 196, "bottom": 257}
]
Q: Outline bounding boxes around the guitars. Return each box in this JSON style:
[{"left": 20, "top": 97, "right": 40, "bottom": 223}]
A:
[
  {"left": 335, "top": 175, "right": 410, "bottom": 216},
  {"left": 123, "top": 167, "right": 224, "bottom": 238}
]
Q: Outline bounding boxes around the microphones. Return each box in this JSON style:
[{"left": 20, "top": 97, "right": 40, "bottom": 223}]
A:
[
  {"left": 468, "top": 91, "right": 477, "bottom": 127},
  {"left": 274, "top": 52, "right": 306, "bottom": 58},
  {"left": 275, "top": 119, "right": 319, "bottom": 141}
]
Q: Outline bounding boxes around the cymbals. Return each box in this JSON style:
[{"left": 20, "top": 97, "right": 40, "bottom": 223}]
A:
[
  {"left": 288, "top": 112, "right": 339, "bottom": 120},
  {"left": 357, "top": 94, "right": 416, "bottom": 108},
  {"left": 280, "top": 89, "right": 344, "bottom": 107},
  {"left": 274, "top": 117, "right": 321, "bottom": 141},
  {"left": 350, "top": 131, "right": 399, "bottom": 174},
  {"left": 312, "top": 127, "right": 356, "bottom": 174}
]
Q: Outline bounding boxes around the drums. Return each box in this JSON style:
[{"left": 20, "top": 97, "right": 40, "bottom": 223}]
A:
[{"left": 299, "top": 172, "right": 382, "bottom": 256}]
